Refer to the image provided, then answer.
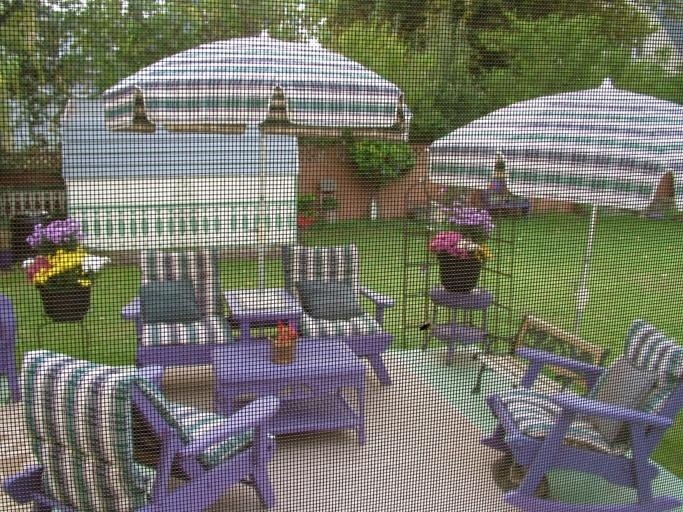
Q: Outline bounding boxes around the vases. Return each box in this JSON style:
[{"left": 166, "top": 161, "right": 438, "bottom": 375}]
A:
[
  {"left": 39, "top": 286, "right": 90, "bottom": 323},
  {"left": 440, "top": 259, "right": 482, "bottom": 292},
  {"left": 272, "top": 340, "right": 296, "bottom": 365}
]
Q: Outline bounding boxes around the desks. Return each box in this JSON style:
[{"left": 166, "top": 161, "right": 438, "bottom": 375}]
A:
[{"left": 224, "top": 287, "right": 303, "bottom": 342}]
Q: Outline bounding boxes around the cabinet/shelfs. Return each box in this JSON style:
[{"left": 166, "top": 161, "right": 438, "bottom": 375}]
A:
[{"left": 421, "top": 287, "right": 493, "bottom": 367}]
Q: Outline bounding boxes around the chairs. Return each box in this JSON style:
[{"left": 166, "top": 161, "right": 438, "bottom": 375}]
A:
[
  {"left": 279, "top": 242, "right": 396, "bottom": 385},
  {"left": 120, "top": 245, "right": 234, "bottom": 366},
  {"left": 483, "top": 317, "right": 683, "bottom": 511},
  {"left": 2, "top": 346, "right": 285, "bottom": 511}
]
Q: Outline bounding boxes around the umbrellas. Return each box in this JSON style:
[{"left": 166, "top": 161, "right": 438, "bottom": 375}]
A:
[
  {"left": 427, "top": 77, "right": 683, "bottom": 335},
  {"left": 100, "top": 25, "right": 415, "bottom": 296}
]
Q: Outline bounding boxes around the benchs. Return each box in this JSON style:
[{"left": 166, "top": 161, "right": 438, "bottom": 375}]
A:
[
  {"left": 480, "top": 180, "right": 531, "bottom": 219},
  {"left": 471, "top": 316, "right": 610, "bottom": 402}
]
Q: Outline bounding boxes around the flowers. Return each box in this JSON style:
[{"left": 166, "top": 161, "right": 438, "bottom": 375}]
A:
[
  {"left": 22, "top": 218, "right": 111, "bottom": 288},
  {"left": 274, "top": 318, "right": 298, "bottom": 342},
  {"left": 431, "top": 200, "right": 495, "bottom": 267}
]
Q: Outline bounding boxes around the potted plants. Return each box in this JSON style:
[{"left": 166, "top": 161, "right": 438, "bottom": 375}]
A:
[{"left": 297, "top": 193, "right": 339, "bottom": 232}]
